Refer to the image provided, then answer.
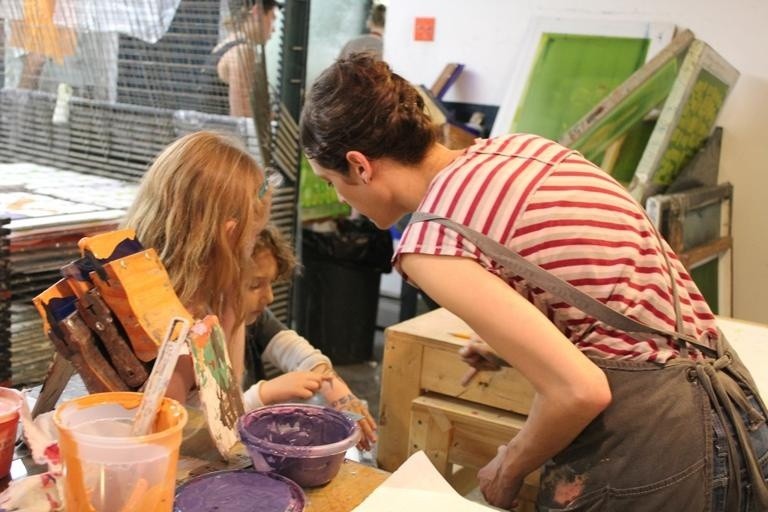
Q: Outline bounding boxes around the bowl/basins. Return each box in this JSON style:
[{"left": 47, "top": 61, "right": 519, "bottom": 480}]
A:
[{"left": 238, "top": 403, "right": 362, "bottom": 488}]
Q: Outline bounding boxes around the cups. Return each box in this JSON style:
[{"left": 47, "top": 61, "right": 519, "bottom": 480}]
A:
[{"left": 0, "top": 386, "right": 23, "bottom": 480}]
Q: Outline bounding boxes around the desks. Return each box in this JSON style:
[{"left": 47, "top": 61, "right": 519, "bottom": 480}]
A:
[
  {"left": 372, "top": 306, "right": 768, "bottom": 512},
  {"left": 304, "top": 460, "right": 502, "bottom": 512}
]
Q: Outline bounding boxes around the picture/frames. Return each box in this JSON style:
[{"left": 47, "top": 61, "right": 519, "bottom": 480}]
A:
[{"left": 489, "top": 18, "right": 740, "bottom": 199}]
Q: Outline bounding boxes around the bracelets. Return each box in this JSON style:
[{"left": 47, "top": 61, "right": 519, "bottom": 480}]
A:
[
  {"left": 326, "top": 394, "right": 358, "bottom": 408},
  {"left": 339, "top": 398, "right": 369, "bottom": 413}
]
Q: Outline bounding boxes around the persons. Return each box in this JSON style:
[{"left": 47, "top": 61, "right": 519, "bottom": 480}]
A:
[
  {"left": 214, "top": 219, "right": 380, "bottom": 460},
  {"left": 299, "top": 46, "right": 766, "bottom": 512},
  {"left": 194, "top": 0, "right": 282, "bottom": 191},
  {"left": 15, "top": 30, "right": 107, "bottom": 163},
  {"left": 112, "top": 130, "right": 274, "bottom": 426},
  {"left": 336, "top": 4, "right": 387, "bottom": 64}
]
